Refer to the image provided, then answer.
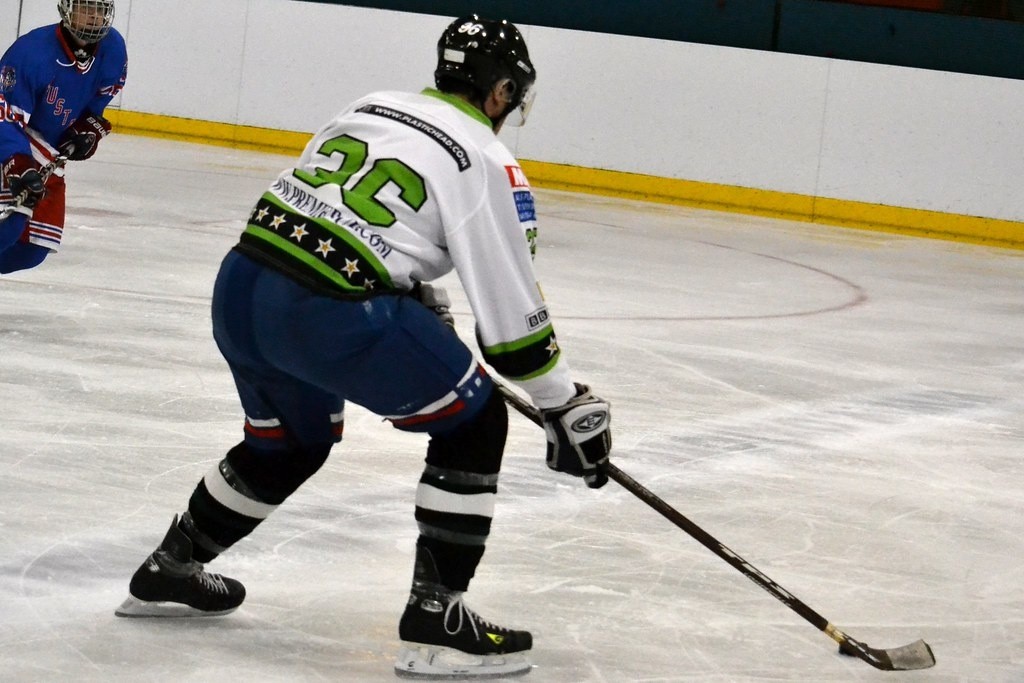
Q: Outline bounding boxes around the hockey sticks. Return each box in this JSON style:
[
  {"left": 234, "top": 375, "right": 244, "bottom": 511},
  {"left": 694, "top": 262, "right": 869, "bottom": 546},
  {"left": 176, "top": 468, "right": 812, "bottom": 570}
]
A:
[
  {"left": 0, "top": 149, "right": 73, "bottom": 217},
  {"left": 491, "top": 378, "right": 937, "bottom": 674}
]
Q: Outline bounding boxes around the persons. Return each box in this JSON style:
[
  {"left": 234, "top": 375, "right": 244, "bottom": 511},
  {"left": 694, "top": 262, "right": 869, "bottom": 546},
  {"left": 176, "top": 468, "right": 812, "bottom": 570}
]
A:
[
  {"left": 113, "top": 9, "right": 613, "bottom": 677},
  {"left": 0, "top": 0, "right": 127, "bottom": 273}
]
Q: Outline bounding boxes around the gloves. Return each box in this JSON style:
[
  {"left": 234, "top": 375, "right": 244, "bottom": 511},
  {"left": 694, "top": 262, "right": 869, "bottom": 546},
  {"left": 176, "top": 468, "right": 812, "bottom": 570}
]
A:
[
  {"left": 2, "top": 153, "right": 47, "bottom": 206},
  {"left": 56, "top": 106, "right": 112, "bottom": 161},
  {"left": 538, "top": 382, "right": 612, "bottom": 488}
]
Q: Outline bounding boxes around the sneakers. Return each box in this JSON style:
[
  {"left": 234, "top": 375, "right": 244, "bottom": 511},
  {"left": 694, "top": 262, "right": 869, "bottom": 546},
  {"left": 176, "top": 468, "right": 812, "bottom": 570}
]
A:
[
  {"left": 114, "top": 512, "right": 246, "bottom": 621},
  {"left": 392, "top": 578, "right": 532, "bottom": 679}
]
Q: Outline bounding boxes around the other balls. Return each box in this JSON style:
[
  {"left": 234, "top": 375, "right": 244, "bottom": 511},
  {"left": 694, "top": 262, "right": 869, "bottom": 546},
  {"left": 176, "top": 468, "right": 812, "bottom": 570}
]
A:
[{"left": 837, "top": 640, "right": 869, "bottom": 657}]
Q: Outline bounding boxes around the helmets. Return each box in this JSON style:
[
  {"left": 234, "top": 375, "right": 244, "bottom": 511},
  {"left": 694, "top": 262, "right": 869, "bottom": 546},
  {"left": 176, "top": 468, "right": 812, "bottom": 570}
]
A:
[
  {"left": 434, "top": 8, "right": 535, "bottom": 115},
  {"left": 58, "top": 0, "right": 115, "bottom": 42}
]
